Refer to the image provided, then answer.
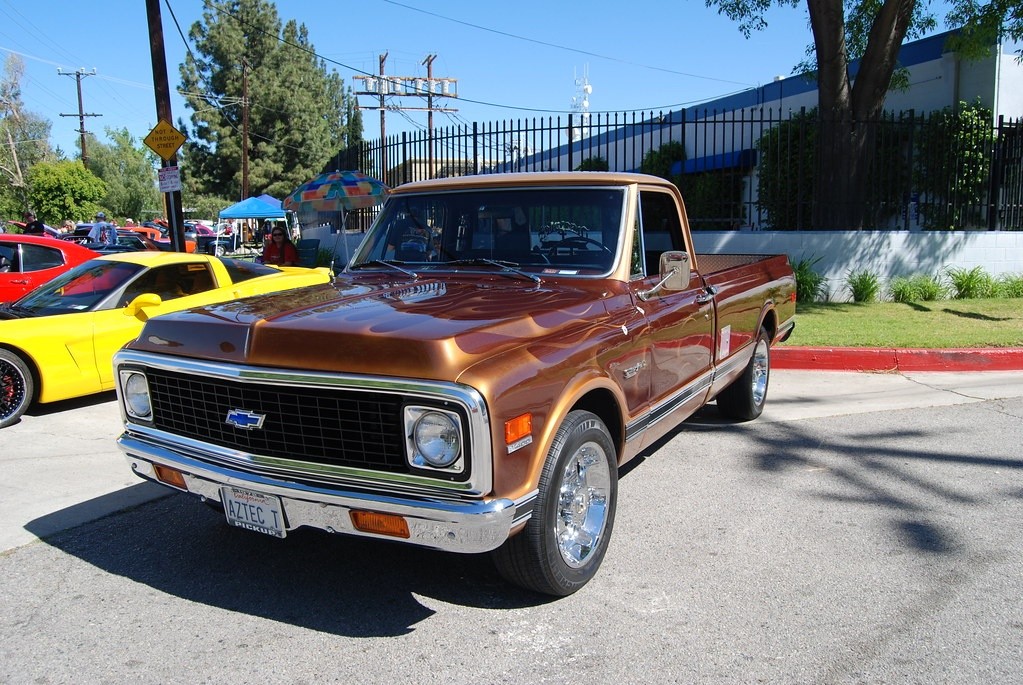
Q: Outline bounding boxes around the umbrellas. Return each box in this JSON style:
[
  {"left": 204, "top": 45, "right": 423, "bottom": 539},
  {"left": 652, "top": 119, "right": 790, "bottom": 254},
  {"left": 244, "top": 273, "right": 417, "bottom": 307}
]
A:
[{"left": 280, "top": 169, "right": 392, "bottom": 266}]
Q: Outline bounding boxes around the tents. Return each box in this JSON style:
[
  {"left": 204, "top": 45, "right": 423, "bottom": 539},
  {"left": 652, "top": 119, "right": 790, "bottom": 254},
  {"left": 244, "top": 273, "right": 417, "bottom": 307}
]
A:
[{"left": 215, "top": 193, "right": 292, "bottom": 257}]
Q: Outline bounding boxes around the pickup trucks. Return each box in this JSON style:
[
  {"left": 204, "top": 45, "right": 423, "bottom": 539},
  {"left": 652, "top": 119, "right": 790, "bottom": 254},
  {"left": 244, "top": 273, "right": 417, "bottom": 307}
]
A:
[{"left": 114, "top": 172, "right": 796, "bottom": 596}]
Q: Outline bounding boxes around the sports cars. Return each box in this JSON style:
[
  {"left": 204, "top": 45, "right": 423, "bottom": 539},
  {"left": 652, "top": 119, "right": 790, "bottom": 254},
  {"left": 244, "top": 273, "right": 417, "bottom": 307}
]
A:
[{"left": 0, "top": 251, "right": 335, "bottom": 429}]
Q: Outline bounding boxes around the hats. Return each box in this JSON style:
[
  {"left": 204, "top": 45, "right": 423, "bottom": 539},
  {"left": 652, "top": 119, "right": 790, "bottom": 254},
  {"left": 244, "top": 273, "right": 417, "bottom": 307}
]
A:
[{"left": 94, "top": 212, "right": 105, "bottom": 219}]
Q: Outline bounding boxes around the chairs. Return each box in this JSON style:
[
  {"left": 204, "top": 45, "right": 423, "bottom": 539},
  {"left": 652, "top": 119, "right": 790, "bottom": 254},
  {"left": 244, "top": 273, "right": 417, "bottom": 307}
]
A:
[{"left": 295, "top": 238, "right": 320, "bottom": 267}]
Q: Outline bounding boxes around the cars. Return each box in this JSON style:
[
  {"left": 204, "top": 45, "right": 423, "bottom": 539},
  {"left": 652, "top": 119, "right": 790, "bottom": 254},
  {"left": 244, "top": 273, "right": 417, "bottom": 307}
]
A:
[
  {"left": 54, "top": 221, "right": 246, "bottom": 258},
  {"left": 0, "top": 234, "right": 136, "bottom": 303}
]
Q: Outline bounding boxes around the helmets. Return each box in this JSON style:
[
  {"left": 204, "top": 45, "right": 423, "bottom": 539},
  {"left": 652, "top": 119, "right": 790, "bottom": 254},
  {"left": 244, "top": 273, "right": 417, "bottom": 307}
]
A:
[{"left": 25, "top": 210, "right": 36, "bottom": 220}]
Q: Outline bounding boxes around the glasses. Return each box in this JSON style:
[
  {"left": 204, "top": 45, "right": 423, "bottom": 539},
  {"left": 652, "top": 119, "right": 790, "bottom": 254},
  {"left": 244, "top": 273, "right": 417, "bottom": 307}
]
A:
[{"left": 272, "top": 233, "right": 283, "bottom": 237}]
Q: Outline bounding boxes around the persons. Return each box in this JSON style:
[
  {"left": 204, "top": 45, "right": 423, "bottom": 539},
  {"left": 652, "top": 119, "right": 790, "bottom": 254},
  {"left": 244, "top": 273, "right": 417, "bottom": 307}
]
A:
[
  {"left": 262, "top": 227, "right": 301, "bottom": 266},
  {"left": 51, "top": 217, "right": 140, "bottom": 251},
  {"left": 153, "top": 216, "right": 161, "bottom": 224},
  {"left": 23, "top": 212, "right": 44, "bottom": 234},
  {"left": 87, "top": 212, "right": 119, "bottom": 249},
  {"left": 227, "top": 219, "right": 241, "bottom": 252}
]
